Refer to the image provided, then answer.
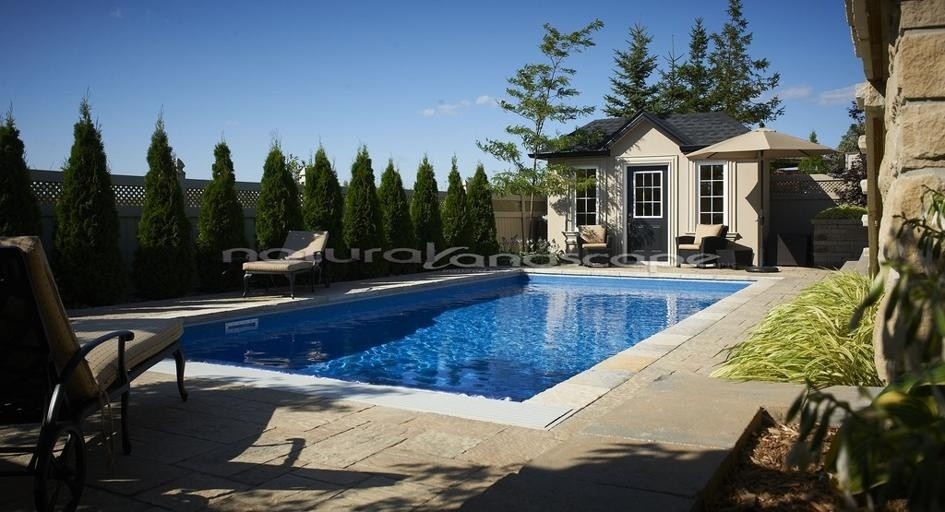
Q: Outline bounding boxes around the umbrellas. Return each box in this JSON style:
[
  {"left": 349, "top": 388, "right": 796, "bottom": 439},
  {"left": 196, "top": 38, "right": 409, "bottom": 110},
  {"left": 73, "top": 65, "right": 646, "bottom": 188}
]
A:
[{"left": 684, "top": 121, "right": 845, "bottom": 268}]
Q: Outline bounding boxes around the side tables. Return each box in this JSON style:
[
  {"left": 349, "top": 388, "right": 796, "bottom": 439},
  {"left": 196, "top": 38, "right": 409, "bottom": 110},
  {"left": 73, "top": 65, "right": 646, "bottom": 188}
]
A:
[{"left": 716, "top": 249, "right": 753, "bottom": 270}]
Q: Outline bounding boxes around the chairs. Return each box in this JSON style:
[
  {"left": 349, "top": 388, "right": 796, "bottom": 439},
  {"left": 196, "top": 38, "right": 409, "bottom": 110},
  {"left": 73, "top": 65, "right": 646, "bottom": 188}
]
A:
[
  {"left": 675, "top": 224, "right": 729, "bottom": 268},
  {"left": 576, "top": 224, "right": 612, "bottom": 268},
  {"left": 241, "top": 230, "right": 330, "bottom": 300},
  {"left": 0, "top": 234, "right": 188, "bottom": 511}
]
